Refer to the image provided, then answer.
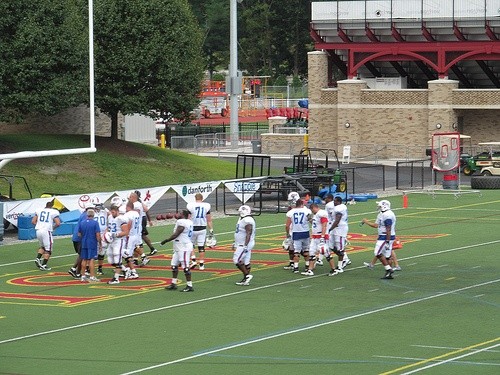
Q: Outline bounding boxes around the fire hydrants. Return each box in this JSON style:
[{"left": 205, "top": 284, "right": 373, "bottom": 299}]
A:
[{"left": 159, "top": 135, "right": 166, "bottom": 150}]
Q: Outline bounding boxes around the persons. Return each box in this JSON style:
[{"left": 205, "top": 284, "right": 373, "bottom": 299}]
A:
[
  {"left": 77, "top": 209, "right": 101, "bottom": 283},
  {"left": 161, "top": 210, "right": 193, "bottom": 292},
  {"left": 363, "top": 200, "right": 401, "bottom": 280},
  {"left": 283, "top": 191, "right": 351, "bottom": 273},
  {"left": 68, "top": 191, "right": 157, "bottom": 279},
  {"left": 31, "top": 202, "right": 60, "bottom": 270},
  {"left": 232, "top": 205, "right": 256, "bottom": 285},
  {"left": 304, "top": 203, "right": 339, "bottom": 277},
  {"left": 106, "top": 206, "right": 130, "bottom": 284},
  {"left": 187, "top": 193, "right": 213, "bottom": 270}
]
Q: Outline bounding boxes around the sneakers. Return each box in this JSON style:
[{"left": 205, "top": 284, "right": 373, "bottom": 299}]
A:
[
  {"left": 334, "top": 266, "right": 344, "bottom": 273},
  {"left": 300, "top": 269, "right": 314, "bottom": 275},
  {"left": 35, "top": 249, "right": 204, "bottom": 292},
  {"left": 293, "top": 267, "right": 299, "bottom": 273},
  {"left": 381, "top": 275, "right": 394, "bottom": 279},
  {"left": 236, "top": 279, "right": 249, "bottom": 286},
  {"left": 284, "top": 263, "right": 294, "bottom": 269},
  {"left": 384, "top": 269, "right": 394, "bottom": 277},
  {"left": 342, "top": 259, "right": 351, "bottom": 269},
  {"left": 244, "top": 274, "right": 253, "bottom": 285},
  {"left": 392, "top": 266, "right": 401, "bottom": 271},
  {"left": 364, "top": 262, "right": 373, "bottom": 269},
  {"left": 329, "top": 269, "right": 337, "bottom": 276},
  {"left": 305, "top": 265, "right": 310, "bottom": 271},
  {"left": 316, "top": 258, "right": 324, "bottom": 266}
]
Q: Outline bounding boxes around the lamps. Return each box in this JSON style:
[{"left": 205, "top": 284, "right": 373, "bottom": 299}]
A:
[
  {"left": 436, "top": 123, "right": 441, "bottom": 129},
  {"left": 345, "top": 122, "right": 350, "bottom": 128},
  {"left": 452, "top": 123, "right": 455, "bottom": 129}
]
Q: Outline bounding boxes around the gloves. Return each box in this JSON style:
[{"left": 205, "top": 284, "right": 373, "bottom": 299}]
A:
[
  {"left": 232, "top": 243, "right": 236, "bottom": 249},
  {"left": 112, "top": 233, "right": 117, "bottom": 238},
  {"left": 243, "top": 246, "right": 248, "bottom": 253}
]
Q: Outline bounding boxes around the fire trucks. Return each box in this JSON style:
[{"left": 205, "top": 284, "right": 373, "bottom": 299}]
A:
[{"left": 188, "top": 92, "right": 229, "bottom": 118}]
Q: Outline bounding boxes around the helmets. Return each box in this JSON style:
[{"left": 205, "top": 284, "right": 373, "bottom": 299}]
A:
[
  {"left": 287, "top": 192, "right": 300, "bottom": 206},
  {"left": 238, "top": 205, "right": 252, "bottom": 217},
  {"left": 377, "top": 199, "right": 391, "bottom": 212},
  {"left": 111, "top": 196, "right": 123, "bottom": 208},
  {"left": 282, "top": 238, "right": 290, "bottom": 251},
  {"left": 206, "top": 237, "right": 216, "bottom": 248}
]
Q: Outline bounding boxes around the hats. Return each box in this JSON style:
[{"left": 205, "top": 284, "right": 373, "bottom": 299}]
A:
[{"left": 85, "top": 203, "right": 95, "bottom": 208}]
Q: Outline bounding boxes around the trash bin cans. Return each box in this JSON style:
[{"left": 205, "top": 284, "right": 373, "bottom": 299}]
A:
[
  {"left": 251, "top": 140, "right": 261, "bottom": 154},
  {"left": 442, "top": 168, "right": 458, "bottom": 189}
]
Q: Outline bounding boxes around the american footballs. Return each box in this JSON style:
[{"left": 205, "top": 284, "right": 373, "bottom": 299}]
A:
[{"left": 155, "top": 213, "right": 180, "bottom": 220}]
